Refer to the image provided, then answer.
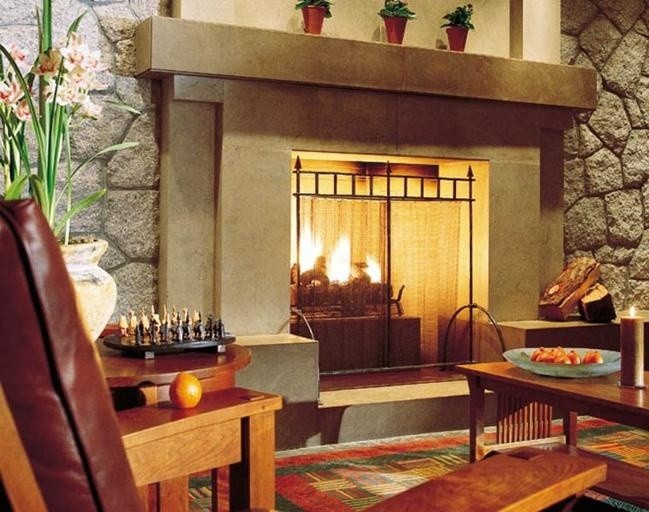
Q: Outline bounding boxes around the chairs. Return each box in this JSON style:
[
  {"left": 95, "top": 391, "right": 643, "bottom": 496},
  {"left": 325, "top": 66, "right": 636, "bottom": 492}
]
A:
[{"left": 1, "top": 198, "right": 608, "bottom": 511}]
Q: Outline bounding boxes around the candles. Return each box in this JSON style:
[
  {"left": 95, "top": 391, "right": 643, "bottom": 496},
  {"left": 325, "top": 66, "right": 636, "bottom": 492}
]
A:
[{"left": 618, "top": 306, "right": 646, "bottom": 388}]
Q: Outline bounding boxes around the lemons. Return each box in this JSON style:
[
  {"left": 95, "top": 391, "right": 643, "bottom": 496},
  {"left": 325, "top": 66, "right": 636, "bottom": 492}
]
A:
[{"left": 169, "top": 373, "right": 203, "bottom": 408}]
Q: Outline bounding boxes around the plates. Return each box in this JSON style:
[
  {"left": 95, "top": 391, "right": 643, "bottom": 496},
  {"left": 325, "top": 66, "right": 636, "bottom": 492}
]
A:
[{"left": 500, "top": 344, "right": 621, "bottom": 380}]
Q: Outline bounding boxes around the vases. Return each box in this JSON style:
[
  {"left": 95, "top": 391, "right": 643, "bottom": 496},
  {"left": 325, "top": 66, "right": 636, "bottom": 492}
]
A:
[{"left": 57, "top": 236, "right": 118, "bottom": 345}]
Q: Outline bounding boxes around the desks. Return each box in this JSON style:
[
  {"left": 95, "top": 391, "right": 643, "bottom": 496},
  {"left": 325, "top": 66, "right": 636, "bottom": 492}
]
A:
[
  {"left": 456, "top": 360, "right": 649, "bottom": 512},
  {"left": 92, "top": 337, "right": 252, "bottom": 511}
]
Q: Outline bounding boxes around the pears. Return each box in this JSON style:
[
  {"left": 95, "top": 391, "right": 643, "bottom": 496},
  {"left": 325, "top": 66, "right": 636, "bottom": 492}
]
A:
[{"left": 530, "top": 346, "right": 603, "bottom": 365}]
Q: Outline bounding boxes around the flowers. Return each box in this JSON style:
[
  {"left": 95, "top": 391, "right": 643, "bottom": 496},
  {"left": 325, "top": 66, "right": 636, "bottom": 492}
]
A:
[{"left": 0, "top": 1, "right": 158, "bottom": 243}]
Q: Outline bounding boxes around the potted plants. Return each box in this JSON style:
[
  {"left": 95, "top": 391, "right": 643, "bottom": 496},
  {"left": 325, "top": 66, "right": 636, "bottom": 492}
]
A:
[
  {"left": 296, "top": 0, "right": 333, "bottom": 36},
  {"left": 440, "top": 3, "right": 477, "bottom": 52},
  {"left": 377, "top": 1, "right": 415, "bottom": 45}
]
